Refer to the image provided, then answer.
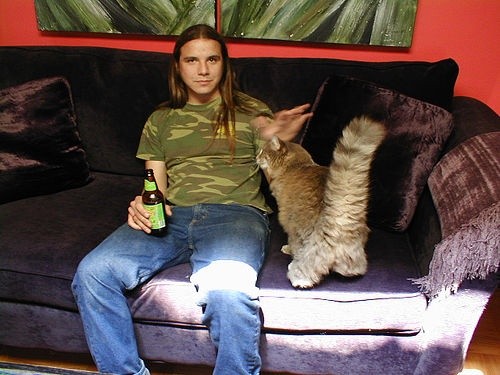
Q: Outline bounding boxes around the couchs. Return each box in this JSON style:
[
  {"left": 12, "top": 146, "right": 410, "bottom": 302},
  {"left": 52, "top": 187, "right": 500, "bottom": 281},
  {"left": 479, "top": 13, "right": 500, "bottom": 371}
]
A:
[{"left": 0, "top": 42, "right": 500, "bottom": 375}]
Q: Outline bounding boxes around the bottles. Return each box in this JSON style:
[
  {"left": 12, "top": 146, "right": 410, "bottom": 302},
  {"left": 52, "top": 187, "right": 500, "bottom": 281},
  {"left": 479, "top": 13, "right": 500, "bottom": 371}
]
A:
[{"left": 142, "top": 168, "right": 168, "bottom": 237}]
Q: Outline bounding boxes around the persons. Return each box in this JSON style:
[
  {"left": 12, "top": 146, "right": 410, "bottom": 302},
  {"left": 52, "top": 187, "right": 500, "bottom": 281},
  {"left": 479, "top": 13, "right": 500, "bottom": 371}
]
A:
[{"left": 69, "top": 22, "right": 314, "bottom": 375}]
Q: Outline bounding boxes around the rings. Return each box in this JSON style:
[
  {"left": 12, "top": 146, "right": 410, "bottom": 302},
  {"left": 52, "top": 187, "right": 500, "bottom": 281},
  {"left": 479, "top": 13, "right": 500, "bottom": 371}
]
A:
[{"left": 132, "top": 214, "right": 136, "bottom": 221}]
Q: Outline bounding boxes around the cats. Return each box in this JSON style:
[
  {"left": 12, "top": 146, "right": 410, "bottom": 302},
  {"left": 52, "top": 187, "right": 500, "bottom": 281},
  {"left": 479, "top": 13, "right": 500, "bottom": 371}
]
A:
[{"left": 255, "top": 115, "right": 389, "bottom": 290}]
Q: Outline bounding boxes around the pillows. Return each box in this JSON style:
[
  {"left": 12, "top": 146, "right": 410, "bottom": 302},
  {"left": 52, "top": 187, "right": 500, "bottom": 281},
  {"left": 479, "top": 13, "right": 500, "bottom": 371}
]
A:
[
  {"left": 1, "top": 77, "right": 95, "bottom": 208},
  {"left": 298, "top": 75, "right": 454, "bottom": 233}
]
